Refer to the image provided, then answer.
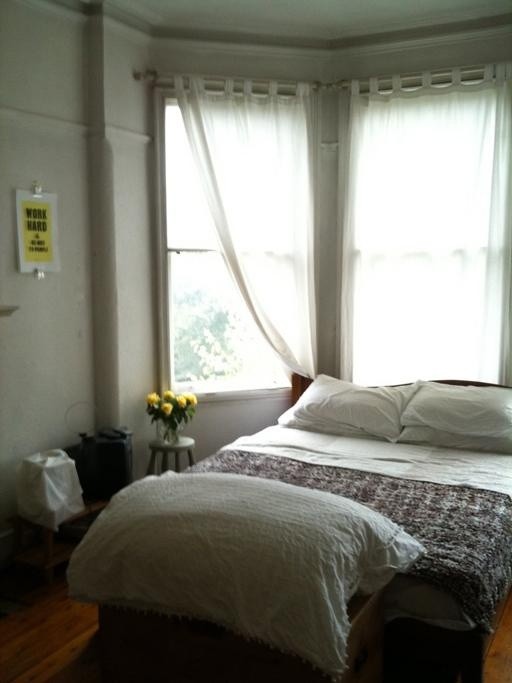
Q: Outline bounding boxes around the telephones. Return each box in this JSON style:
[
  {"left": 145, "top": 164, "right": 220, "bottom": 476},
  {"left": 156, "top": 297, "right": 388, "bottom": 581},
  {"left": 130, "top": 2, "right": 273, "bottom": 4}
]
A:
[{"left": 100, "top": 429, "right": 133, "bottom": 439}]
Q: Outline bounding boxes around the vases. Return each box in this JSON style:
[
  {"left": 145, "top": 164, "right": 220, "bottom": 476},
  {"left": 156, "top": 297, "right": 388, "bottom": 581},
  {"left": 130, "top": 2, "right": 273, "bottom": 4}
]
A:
[{"left": 160, "top": 422, "right": 181, "bottom": 446}]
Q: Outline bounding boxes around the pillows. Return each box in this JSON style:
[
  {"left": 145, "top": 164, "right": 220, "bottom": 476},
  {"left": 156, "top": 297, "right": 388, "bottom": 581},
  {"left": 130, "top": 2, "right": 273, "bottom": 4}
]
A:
[
  {"left": 399, "top": 381, "right": 512, "bottom": 454},
  {"left": 278, "top": 373, "right": 425, "bottom": 447}
]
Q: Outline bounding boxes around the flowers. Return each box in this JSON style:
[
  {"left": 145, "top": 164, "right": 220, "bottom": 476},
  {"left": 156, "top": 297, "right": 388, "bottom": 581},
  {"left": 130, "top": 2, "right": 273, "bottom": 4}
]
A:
[{"left": 146, "top": 389, "right": 198, "bottom": 446}]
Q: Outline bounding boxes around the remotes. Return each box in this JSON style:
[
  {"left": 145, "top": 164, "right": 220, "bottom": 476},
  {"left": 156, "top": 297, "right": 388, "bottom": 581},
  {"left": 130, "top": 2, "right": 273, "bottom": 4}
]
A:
[
  {"left": 99, "top": 430, "right": 120, "bottom": 439},
  {"left": 112, "top": 428, "right": 127, "bottom": 438}
]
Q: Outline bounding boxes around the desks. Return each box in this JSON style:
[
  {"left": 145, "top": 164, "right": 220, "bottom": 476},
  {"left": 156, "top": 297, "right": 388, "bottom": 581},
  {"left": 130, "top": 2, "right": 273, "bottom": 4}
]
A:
[{"left": 10, "top": 503, "right": 111, "bottom": 585}]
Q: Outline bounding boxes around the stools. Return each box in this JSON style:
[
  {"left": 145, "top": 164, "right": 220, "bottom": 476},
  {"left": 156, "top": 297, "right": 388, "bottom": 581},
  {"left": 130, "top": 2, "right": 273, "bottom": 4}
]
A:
[
  {"left": 98, "top": 591, "right": 386, "bottom": 683},
  {"left": 145, "top": 436, "right": 196, "bottom": 476}
]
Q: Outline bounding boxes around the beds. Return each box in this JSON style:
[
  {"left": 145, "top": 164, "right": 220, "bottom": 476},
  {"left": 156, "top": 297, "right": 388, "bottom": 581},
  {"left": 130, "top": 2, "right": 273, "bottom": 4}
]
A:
[{"left": 186, "top": 371, "right": 512, "bottom": 682}]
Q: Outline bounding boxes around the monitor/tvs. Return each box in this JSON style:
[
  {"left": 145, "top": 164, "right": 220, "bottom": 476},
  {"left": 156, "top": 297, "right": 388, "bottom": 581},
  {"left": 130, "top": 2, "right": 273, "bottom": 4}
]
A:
[{"left": 79, "top": 432, "right": 133, "bottom": 504}]
